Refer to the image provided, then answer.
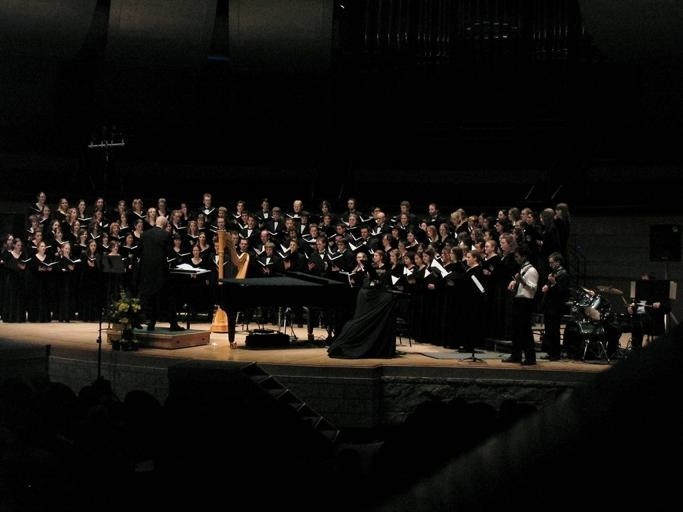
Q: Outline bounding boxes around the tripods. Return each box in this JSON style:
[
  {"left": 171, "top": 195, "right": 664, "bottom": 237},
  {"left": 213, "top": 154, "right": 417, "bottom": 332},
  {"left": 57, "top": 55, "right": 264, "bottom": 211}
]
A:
[
  {"left": 277, "top": 307, "right": 298, "bottom": 341},
  {"left": 663, "top": 263, "right": 679, "bottom": 333},
  {"left": 459, "top": 292, "right": 486, "bottom": 363}
]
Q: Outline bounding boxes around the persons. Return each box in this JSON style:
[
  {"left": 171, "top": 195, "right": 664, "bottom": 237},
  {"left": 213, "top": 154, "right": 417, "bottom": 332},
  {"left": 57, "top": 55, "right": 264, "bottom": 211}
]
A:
[
  {"left": 1, "top": 192, "right": 570, "bottom": 365},
  {"left": 607, "top": 273, "right": 672, "bottom": 358}
]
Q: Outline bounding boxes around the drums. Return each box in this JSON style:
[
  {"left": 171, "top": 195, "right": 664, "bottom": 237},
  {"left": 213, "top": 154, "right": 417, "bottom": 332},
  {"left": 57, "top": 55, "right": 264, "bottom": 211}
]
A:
[
  {"left": 563, "top": 320, "right": 610, "bottom": 362},
  {"left": 576, "top": 294, "right": 610, "bottom": 320}
]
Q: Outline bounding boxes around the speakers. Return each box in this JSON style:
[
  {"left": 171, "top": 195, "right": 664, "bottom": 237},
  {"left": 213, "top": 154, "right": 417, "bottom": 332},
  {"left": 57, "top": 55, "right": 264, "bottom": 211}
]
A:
[{"left": 648, "top": 224, "right": 682, "bottom": 263}]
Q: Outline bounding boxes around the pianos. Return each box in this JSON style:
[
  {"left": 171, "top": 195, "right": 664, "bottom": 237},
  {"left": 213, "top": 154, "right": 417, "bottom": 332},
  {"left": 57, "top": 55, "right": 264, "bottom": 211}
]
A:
[{"left": 216, "top": 271, "right": 350, "bottom": 343}]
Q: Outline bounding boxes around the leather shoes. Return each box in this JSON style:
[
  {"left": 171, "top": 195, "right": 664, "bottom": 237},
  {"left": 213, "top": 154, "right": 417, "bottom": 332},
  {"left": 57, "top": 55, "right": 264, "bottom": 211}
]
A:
[
  {"left": 147, "top": 323, "right": 155, "bottom": 329},
  {"left": 170, "top": 323, "right": 186, "bottom": 331},
  {"left": 501, "top": 355, "right": 537, "bottom": 366},
  {"left": 541, "top": 354, "right": 561, "bottom": 361}
]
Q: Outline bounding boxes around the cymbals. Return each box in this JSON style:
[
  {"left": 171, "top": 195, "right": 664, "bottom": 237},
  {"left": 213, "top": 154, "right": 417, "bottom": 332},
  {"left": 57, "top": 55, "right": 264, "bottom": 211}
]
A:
[
  {"left": 597, "top": 286, "right": 622, "bottom": 294},
  {"left": 582, "top": 288, "right": 598, "bottom": 298}
]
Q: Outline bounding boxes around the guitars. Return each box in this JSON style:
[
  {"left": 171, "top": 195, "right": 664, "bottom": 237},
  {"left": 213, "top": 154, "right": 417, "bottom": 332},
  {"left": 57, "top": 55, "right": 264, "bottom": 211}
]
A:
[
  {"left": 539, "top": 264, "right": 559, "bottom": 302},
  {"left": 510, "top": 256, "right": 525, "bottom": 297}
]
